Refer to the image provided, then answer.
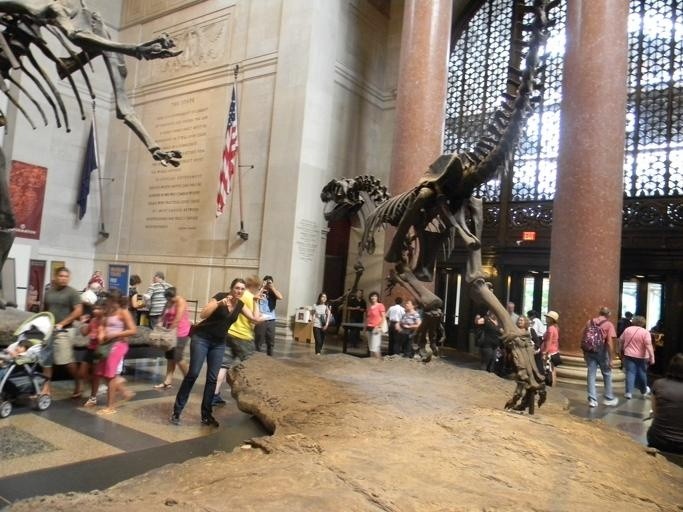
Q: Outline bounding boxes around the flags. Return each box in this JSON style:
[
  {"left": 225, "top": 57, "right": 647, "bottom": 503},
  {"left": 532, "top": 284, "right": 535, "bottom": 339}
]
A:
[{"left": 212, "top": 84, "right": 238, "bottom": 221}]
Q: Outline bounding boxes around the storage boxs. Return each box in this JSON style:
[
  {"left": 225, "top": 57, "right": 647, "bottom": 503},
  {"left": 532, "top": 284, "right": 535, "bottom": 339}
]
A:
[{"left": 294, "top": 309, "right": 314, "bottom": 323}]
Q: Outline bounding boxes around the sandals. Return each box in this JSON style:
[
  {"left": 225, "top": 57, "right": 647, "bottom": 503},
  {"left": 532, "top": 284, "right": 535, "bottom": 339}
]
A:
[
  {"left": 96, "top": 407, "right": 117, "bottom": 416},
  {"left": 153, "top": 382, "right": 173, "bottom": 390}
]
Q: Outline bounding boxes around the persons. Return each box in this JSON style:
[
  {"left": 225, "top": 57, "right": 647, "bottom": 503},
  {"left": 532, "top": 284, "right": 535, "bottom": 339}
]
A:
[
  {"left": 170, "top": 279, "right": 263, "bottom": 428},
  {"left": 212, "top": 275, "right": 282, "bottom": 405},
  {"left": 620, "top": 316, "right": 655, "bottom": 398},
  {"left": 1, "top": 269, "right": 192, "bottom": 415},
  {"left": 308, "top": 293, "right": 332, "bottom": 357},
  {"left": 473, "top": 301, "right": 562, "bottom": 388},
  {"left": 645, "top": 353, "right": 683, "bottom": 454},
  {"left": 619, "top": 309, "right": 632, "bottom": 330},
  {"left": 348, "top": 289, "right": 421, "bottom": 363},
  {"left": 579, "top": 305, "right": 619, "bottom": 408}
]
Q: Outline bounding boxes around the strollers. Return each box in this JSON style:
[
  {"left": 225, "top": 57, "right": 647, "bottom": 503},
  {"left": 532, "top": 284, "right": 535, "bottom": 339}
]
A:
[{"left": 0, "top": 307, "right": 71, "bottom": 421}]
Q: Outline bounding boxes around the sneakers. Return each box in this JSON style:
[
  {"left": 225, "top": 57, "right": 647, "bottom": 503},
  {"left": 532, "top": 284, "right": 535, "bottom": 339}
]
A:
[
  {"left": 201, "top": 414, "right": 219, "bottom": 429},
  {"left": 211, "top": 395, "right": 226, "bottom": 406},
  {"left": 168, "top": 412, "right": 183, "bottom": 425},
  {"left": 118, "top": 391, "right": 137, "bottom": 407},
  {"left": 604, "top": 397, "right": 619, "bottom": 406},
  {"left": 624, "top": 392, "right": 633, "bottom": 399},
  {"left": 588, "top": 399, "right": 599, "bottom": 408},
  {"left": 642, "top": 386, "right": 650, "bottom": 399},
  {"left": 81, "top": 396, "right": 97, "bottom": 408},
  {"left": 69, "top": 391, "right": 81, "bottom": 402}
]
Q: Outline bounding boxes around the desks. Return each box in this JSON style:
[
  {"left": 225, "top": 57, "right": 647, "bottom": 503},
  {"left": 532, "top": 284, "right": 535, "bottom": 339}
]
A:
[
  {"left": 294, "top": 322, "right": 316, "bottom": 344},
  {"left": 342, "top": 322, "right": 368, "bottom": 353}
]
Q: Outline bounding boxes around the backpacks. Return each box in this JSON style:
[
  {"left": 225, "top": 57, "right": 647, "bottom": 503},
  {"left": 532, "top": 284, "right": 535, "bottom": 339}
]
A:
[{"left": 580, "top": 318, "right": 609, "bottom": 354}]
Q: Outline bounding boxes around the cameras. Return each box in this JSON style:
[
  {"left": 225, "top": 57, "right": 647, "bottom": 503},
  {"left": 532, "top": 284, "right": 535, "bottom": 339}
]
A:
[{"left": 267, "top": 281, "right": 271, "bottom": 284}]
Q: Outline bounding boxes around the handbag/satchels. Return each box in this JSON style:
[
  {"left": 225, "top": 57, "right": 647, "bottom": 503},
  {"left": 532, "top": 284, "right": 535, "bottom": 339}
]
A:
[
  {"left": 550, "top": 351, "right": 562, "bottom": 367},
  {"left": 320, "top": 304, "right": 336, "bottom": 327},
  {"left": 93, "top": 337, "right": 124, "bottom": 361}
]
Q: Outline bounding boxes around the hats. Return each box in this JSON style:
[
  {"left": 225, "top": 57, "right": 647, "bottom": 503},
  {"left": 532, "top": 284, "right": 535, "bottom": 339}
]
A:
[{"left": 543, "top": 310, "right": 560, "bottom": 325}]
